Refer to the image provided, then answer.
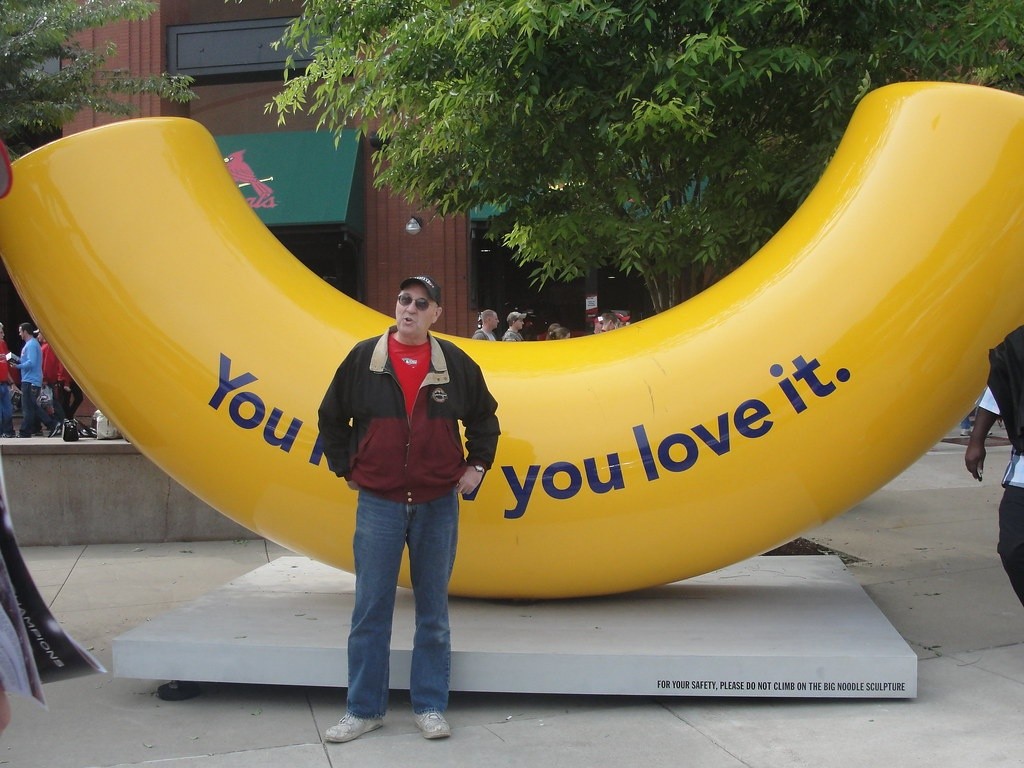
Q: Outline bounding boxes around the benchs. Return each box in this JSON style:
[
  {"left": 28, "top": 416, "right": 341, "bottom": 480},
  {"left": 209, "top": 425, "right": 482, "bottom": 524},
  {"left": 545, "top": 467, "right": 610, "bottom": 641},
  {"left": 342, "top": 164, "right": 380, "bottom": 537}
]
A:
[{"left": 0, "top": 439, "right": 265, "bottom": 547}]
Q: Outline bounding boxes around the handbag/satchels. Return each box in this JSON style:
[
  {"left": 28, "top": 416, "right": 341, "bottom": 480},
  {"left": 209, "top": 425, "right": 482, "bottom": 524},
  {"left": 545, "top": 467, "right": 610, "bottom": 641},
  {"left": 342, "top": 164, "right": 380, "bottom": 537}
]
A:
[
  {"left": 62, "top": 417, "right": 79, "bottom": 441},
  {"left": 93, "top": 410, "right": 122, "bottom": 439},
  {"left": 36, "top": 384, "right": 54, "bottom": 408},
  {"left": 6, "top": 385, "right": 24, "bottom": 410}
]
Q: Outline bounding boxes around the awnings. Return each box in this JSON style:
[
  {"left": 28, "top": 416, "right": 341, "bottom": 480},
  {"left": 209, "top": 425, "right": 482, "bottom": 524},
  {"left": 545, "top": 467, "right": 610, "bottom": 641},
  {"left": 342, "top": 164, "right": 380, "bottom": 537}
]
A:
[
  {"left": 213, "top": 131, "right": 367, "bottom": 244},
  {"left": 468, "top": 162, "right": 710, "bottom": 241}
]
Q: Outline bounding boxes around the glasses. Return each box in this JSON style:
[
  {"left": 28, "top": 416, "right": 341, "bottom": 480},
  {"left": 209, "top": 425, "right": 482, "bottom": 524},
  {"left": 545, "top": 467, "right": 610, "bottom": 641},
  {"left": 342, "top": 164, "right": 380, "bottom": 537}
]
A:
[{"left": 398, "top": 293, "right": 429, "bottom": 311}]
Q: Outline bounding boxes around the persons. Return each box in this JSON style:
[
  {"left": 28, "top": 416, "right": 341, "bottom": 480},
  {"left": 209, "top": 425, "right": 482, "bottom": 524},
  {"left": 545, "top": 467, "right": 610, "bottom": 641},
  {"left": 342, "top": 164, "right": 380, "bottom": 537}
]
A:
[
  {"left": 318, "top": 272, "right": 501, "bottom": 743},
  {"left": 960, "top": 324, "right": 1024, "bottom": 604},
  {"left": 472, "top": 309, "right": 632, "bottom": 342},
  {"left": 0, "top": 322, "right": 84, "bottom": 439}
]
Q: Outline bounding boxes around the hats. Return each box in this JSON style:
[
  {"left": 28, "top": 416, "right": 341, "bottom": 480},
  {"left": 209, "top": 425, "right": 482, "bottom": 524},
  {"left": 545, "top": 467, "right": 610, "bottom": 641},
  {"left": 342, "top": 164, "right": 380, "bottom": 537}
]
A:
[
  {"left": 33, "top": 329, "right": 39, "bottom": 333},
  {"left": 507, "top": 312, "right": 527, "bottom": 325},
  {"left": 400, "top": 274, "right": 441, "bottom": 306}
]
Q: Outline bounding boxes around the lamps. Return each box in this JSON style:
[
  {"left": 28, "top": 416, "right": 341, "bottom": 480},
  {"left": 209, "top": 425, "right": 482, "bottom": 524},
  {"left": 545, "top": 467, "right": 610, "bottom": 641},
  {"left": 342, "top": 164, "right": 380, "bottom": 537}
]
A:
[{"left": 405, "top": 215, "right": 423, "bottom": 236}]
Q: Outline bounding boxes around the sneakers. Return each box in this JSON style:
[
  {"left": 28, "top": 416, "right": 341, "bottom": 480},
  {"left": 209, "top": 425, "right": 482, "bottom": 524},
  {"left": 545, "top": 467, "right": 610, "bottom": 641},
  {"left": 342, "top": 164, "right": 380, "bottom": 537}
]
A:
[
  {"left": 324, "top": 713, "right": 384, "bottom": 742},
  {"left": 960, "top": 429, "right": 968, "bottom": 435},
  {"left": 988, "top": 431, "right": 992, "bottom": 435},
  {"left": 13, "top": 434, "right": 31, "bottom": 438},
  {"left": 413, "top": 709, "right": 451, "bottom": 739},
  {"left": 48, "top": 422, "right": 61, "bottom": 437}
]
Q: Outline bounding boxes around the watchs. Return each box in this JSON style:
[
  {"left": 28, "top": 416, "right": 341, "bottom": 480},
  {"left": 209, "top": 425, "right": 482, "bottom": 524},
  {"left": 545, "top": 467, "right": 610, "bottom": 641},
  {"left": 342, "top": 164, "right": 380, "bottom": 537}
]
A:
[{"left": 473, "top": 464, "right": 485, "bottom": 474}]
